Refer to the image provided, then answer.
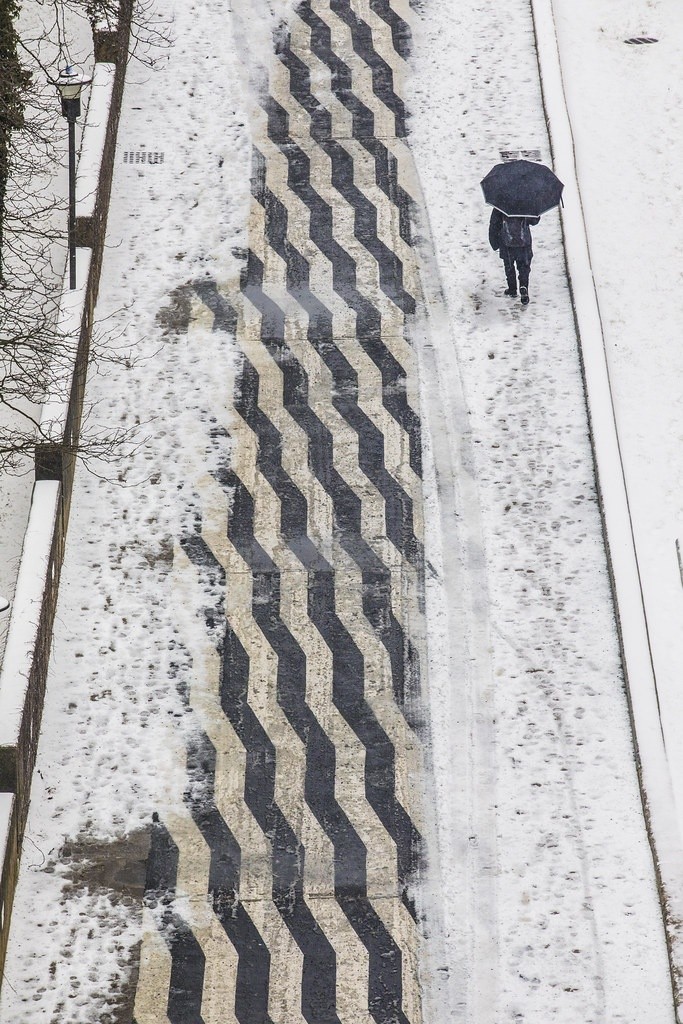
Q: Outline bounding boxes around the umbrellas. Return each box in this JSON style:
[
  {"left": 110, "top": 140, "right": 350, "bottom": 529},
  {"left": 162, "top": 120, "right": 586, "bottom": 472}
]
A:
[{"left": 479, "top": 159, "right": 566, "bottom": 218}]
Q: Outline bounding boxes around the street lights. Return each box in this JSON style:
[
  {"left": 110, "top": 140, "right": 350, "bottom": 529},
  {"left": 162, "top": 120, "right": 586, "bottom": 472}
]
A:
[{"left": 45, "top": 68, "right": 95, "bottom": 295}]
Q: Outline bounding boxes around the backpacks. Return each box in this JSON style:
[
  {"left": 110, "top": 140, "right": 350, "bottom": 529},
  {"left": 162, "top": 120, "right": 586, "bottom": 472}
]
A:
[{"left": 502, "top": 216, "right": 530, "bottom": 247}]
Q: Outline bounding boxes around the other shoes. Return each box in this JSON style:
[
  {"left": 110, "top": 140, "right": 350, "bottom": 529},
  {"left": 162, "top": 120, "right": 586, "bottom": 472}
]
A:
[
  {"left": 519, "top": 286, "right": 529, "bottom": 306},
  {"left": 505, "top": 289, "right": 517, "bottom": 297}
]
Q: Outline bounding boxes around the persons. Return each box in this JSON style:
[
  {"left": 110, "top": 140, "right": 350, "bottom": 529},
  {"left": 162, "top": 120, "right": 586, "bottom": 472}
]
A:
[{"left": 488, "top": 206, "right": 540, "bottom": 305}]
